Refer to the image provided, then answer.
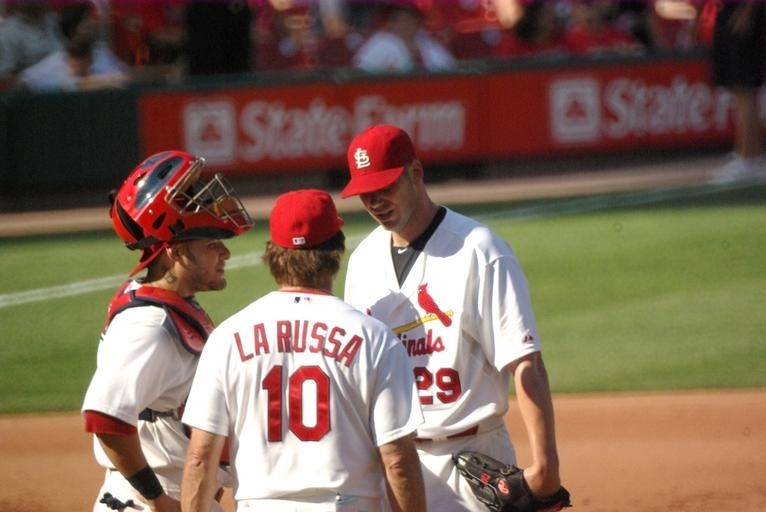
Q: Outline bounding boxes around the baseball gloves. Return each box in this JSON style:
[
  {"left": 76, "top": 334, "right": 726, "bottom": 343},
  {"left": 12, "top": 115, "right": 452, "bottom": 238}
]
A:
[{"left": 456, "top": 450, "right": 572, "bottom": 509}]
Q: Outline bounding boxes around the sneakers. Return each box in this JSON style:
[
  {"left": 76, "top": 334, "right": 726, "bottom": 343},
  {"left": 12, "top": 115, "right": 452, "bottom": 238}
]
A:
[{"left": 712, "top": 153, "right": 764, "bottom": 184}]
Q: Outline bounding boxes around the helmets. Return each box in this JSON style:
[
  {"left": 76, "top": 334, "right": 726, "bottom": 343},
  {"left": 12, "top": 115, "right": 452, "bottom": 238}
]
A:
[{"left": 110, "top": 147, "right": 253, "bottom": 250}]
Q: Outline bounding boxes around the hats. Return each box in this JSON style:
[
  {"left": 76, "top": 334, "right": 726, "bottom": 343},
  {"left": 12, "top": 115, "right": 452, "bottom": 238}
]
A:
[
  {"left": 268, "top": 189, "right": 347, "bottom": 250},
  {"left": 340, "top": 123, "right": 418, "bottom": 199}
]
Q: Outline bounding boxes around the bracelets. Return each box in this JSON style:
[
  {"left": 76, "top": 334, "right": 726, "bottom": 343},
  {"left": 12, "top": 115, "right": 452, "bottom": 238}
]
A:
[{"left": 126, "top": 465, "right": 164, "bottom": 499}]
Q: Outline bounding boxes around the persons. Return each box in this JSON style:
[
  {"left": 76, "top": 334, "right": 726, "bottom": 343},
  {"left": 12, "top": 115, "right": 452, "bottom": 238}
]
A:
[
  {"left": 0, "top": 0, "right": 131, "bottom": 92},
  {"left": 704, "top": 0, "right": 766, "bottom": 184},
  {"left": 180, "top": 188, "right": 426, "bottom": 512},
  {"left": 494, "top": 0, "right": 636, "bottom": 61},
  {"left": 340, "top": 124, "right": 569, "bottom": 512},
  {"left": 80, "top": 150, "right": 255, "bottom": 512},
  {"left": 251, "top": 1, "right": 322, "bottom": 73},
  {"left": 355, "top": 6, "right": 454, "bottom": 73}
]
